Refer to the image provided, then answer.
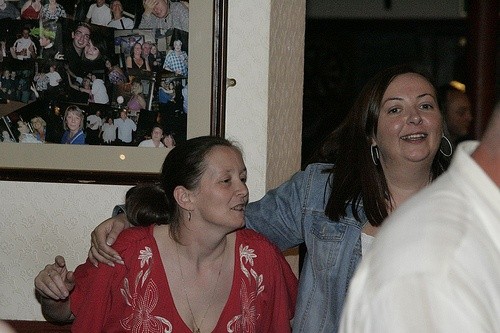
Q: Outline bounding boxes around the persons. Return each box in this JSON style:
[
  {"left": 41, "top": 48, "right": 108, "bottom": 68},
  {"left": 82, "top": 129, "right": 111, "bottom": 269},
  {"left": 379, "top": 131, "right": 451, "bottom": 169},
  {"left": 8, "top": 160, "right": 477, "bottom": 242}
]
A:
[
  {"left": 0, "top": 0, "right": 189, "bottom": 148},
  {"left": 338, "top": 99, "right": 500, "bottom": 333},
  {"left": 88, "top": 64, "right": 454, "bottom": 333},
  {"left": 33, "top": 136, "right": 298, "bottom": 333},
  {"left": 436, "top": 82, "right": 471, "bottom": 175}
]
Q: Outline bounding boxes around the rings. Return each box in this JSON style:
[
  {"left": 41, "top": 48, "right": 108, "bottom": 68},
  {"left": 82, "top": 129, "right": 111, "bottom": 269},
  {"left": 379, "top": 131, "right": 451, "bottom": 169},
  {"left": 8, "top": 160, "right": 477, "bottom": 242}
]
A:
[
  {"left": 48, "top": 269, "right": 57, "bottom": 277},
  {"left": 90, "top": 245, "right": 95, "bottom": 250}
]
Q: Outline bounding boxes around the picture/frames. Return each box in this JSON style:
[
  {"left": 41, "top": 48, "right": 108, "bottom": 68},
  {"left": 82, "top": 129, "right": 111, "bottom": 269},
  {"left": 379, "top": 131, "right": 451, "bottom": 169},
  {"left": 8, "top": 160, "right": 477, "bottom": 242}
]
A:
[{"left": 0, "top": 0, "right": 229, "bottom": 186}]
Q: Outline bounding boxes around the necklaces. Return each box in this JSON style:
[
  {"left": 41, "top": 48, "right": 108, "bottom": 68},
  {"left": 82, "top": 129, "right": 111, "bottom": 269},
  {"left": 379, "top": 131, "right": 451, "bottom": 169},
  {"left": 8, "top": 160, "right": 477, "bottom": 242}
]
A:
[{"left": 175, "top": 239, "right": 225, "bottom": 331}]
